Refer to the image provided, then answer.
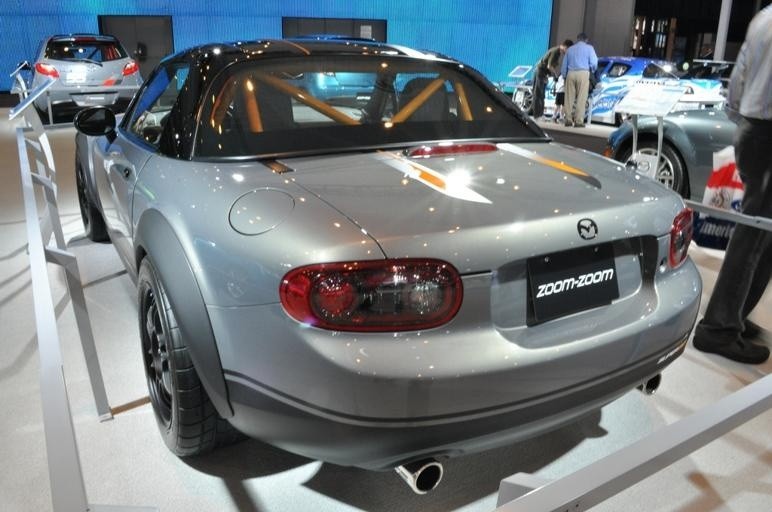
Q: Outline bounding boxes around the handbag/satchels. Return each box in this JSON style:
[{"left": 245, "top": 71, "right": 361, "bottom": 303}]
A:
[{"left": 690, "top": 144, "right": 747, "bottom": 250}]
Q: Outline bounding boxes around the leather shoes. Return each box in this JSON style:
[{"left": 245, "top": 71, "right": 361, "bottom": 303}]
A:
[{"left": 693, "top": 317, "right": 770, "bottom": 364}]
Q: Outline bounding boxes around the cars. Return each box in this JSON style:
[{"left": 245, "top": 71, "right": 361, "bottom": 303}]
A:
[
  {"left": 17, "top": 32, "right": 147, "bottom": 125},
  {"left": 73, "top": 34, "right": 703, "bottom": 497},
  {"left": 604, "top": 109, "right": 745, "bottom": 251}
]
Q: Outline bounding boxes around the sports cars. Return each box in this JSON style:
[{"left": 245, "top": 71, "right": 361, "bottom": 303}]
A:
[{"left": 513, "top": 55, "right": 737, "bottom": 128}]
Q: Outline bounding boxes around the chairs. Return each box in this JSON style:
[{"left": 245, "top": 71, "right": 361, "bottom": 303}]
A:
[
  {"left": 232, "top": 82, "right": 296, "bottom": 133},
  {"left": 398, "top": 77, "right": 450, "bottom": 124}
]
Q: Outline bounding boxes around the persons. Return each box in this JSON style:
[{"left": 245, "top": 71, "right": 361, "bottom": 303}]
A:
[
  {"left": 689, "top": 5, "right": 771, "bottom": 366},
  {"left": 559, "top": 33, "right": 599, "bottom": 129},
  {"left": 532, "top": 40, "right": 574, "bottom": 122},
  {"left": 551, "top": 73, "right": 569, "bottom": 124}
]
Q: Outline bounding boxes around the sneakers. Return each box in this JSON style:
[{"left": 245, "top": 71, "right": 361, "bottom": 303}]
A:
[{"left": 535, "top": 114, "right": 586, "bottom": 128}]
